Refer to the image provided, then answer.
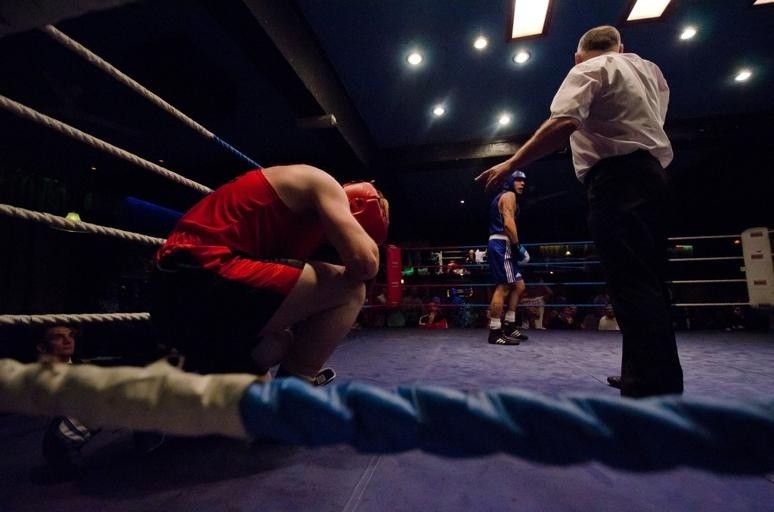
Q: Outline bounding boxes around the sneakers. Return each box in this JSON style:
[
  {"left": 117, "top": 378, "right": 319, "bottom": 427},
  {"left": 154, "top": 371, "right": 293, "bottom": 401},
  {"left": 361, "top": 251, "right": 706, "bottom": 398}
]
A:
[
  {"left": 489, "top": 327, "right": 520, "bottom": 345},
  {"left": 503, "top": 321, "right": 528, "bottom": 339},
  {"left": 43, "top": 416, "right": 100, "bottom": 476}
]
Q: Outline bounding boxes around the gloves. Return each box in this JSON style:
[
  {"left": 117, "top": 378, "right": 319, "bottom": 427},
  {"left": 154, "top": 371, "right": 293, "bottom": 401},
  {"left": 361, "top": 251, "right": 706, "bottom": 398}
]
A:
[{"left": 513, "top": 243, "right": 530, "bottom": 265}]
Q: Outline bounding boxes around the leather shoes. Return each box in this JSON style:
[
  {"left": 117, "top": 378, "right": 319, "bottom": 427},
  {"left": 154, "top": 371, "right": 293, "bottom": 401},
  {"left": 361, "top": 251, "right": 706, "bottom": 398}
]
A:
[{"left": 607, "top": 376, "right": 621, "bottom": 388}]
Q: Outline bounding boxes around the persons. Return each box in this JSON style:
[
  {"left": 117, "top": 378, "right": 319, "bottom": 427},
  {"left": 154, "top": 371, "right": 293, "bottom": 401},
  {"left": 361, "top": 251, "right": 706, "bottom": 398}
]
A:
[
  {"left": 473, "top": 24, "right": 688, "bottom": 400},
  {"left": 143, "top": 338, "right": 187, "bottom": 370},
  {"left": 353, "top": 250, "right": 620, "bottom": 331},
  {"left": 484, "top": 169, "right": 530, "bottom": 346},
  {"left": 155, "top": 162, "right": 389, "bottom": 387},
  {"left": 30, "top": 321, "right": 92, "bottom": 454}
]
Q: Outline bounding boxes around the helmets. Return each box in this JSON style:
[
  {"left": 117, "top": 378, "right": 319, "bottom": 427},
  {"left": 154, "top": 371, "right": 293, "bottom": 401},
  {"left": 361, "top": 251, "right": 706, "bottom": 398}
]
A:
[
  {"left": 503, "top": 171, "right": 527, "bottom": 194},
  {"left": 344, "top": 180, "right": 387, "bottom": 244}
]
